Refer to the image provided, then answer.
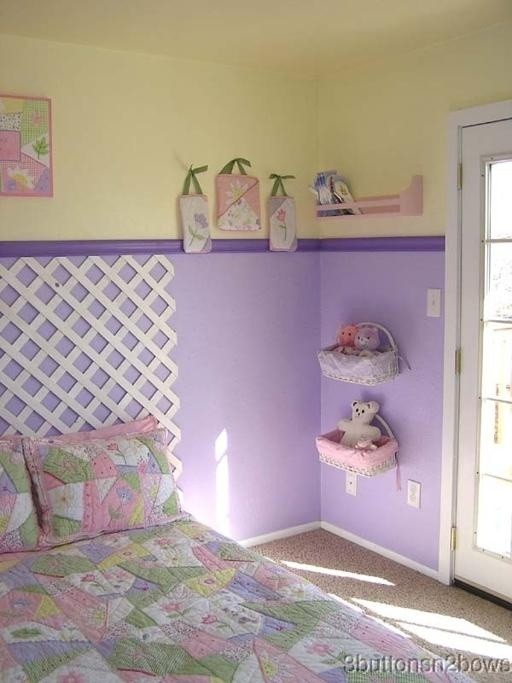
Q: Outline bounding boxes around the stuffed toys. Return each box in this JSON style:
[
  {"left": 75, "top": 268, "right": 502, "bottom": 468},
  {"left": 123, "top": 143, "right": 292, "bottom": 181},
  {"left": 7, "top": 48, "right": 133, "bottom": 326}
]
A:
[
  {"left": 347, "top": 323, "right": 382, "bottom": 356},
  {"left": 334, "top": 399, "right": 381, "bottom": 449},
  {"left": 353, "top": 433, "right": 379, "bottom": 449},
  {"left": 327, "top": 322, "right": 361, "bottom": 355}
]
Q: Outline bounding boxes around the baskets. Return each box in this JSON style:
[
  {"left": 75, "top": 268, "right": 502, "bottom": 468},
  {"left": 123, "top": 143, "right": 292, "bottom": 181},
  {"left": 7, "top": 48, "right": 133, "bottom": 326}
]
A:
[{"left": 314, "top": 413, "right": 397, "bottom": 478}]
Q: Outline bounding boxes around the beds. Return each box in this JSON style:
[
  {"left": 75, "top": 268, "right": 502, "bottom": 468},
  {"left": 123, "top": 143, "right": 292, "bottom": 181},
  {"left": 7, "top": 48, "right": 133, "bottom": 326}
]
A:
[{"left": 1, "top": 413, "right": 483, "bottom": 683}]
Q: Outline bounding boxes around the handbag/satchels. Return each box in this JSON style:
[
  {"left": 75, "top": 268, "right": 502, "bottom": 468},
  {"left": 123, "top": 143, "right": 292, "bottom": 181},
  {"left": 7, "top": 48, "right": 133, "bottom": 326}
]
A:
[
  {"left": 178, "top": 168, "right": 214, "bottom": 254},
  {"left": 214, "top": 157, "right": 264, "bottom": 232},
  {"left": 267, "top": 173, "right": 300, "bottom": 254}
]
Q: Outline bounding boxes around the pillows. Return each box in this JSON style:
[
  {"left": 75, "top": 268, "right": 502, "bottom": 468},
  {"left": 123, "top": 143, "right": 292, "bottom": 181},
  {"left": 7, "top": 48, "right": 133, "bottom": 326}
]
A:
[{"left": 0, "top": 414, "right": 192, "bottom": 555}]
